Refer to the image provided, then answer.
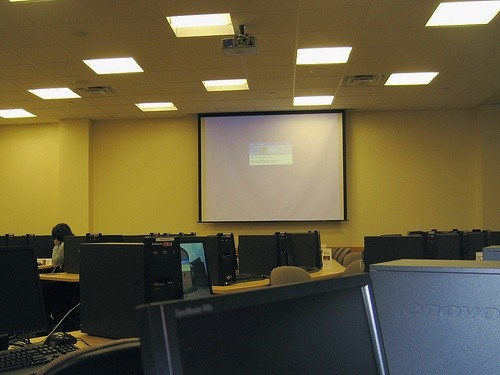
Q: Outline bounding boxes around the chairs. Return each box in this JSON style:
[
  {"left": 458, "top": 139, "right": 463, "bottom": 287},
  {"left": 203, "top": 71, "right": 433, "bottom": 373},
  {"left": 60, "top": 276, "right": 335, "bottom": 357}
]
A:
[{"left": 35, "top": 245, "right": 366, "bottom": 375}]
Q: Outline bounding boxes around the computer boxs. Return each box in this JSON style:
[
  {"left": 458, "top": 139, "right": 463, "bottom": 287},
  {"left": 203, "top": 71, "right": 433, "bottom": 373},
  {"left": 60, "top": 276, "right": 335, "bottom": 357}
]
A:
[{"left": 0, "top": 229, "right": 500, "bottom": 375}]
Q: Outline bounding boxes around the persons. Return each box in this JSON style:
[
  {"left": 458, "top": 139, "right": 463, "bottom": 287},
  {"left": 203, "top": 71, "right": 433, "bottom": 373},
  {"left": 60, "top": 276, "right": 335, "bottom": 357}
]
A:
[{"left": 52, "top": 223, "right": 76, "bottom": 273}]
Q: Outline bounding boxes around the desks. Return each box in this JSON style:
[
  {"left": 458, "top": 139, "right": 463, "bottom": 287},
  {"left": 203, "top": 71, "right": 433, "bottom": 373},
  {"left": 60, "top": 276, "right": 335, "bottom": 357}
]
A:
[{"left": 0, "top": 253, "right": 347, "bottom": 375}]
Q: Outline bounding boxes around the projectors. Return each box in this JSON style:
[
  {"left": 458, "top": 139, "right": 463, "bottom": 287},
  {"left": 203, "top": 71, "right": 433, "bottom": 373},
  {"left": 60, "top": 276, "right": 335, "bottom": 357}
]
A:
[{"left": 220, "top": 34, "right": 260, "bottom": 57}]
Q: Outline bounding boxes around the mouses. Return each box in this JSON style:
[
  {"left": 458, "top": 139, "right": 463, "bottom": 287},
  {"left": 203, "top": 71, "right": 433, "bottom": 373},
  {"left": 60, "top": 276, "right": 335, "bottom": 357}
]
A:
[{"left": 46, "top": 333, "right": 77, "bottom": 344}]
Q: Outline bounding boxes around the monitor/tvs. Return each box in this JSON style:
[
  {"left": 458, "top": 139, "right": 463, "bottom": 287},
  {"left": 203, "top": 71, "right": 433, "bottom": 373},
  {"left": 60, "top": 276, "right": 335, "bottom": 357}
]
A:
[
  {"left": 0, "top": 246, "right": 48, "bottom": 330},
  {"left": 137, "top": 272, "right": 388, "bottom": 375},
  {"left": 177, "top": 236, "right": 213, "bottom": 299}
]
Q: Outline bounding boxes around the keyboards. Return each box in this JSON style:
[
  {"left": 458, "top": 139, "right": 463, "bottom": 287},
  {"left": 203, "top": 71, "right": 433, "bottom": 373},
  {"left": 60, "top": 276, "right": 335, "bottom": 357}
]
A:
[{"left": 0, "top": 342, "right": 77, "bottom": 371}]
